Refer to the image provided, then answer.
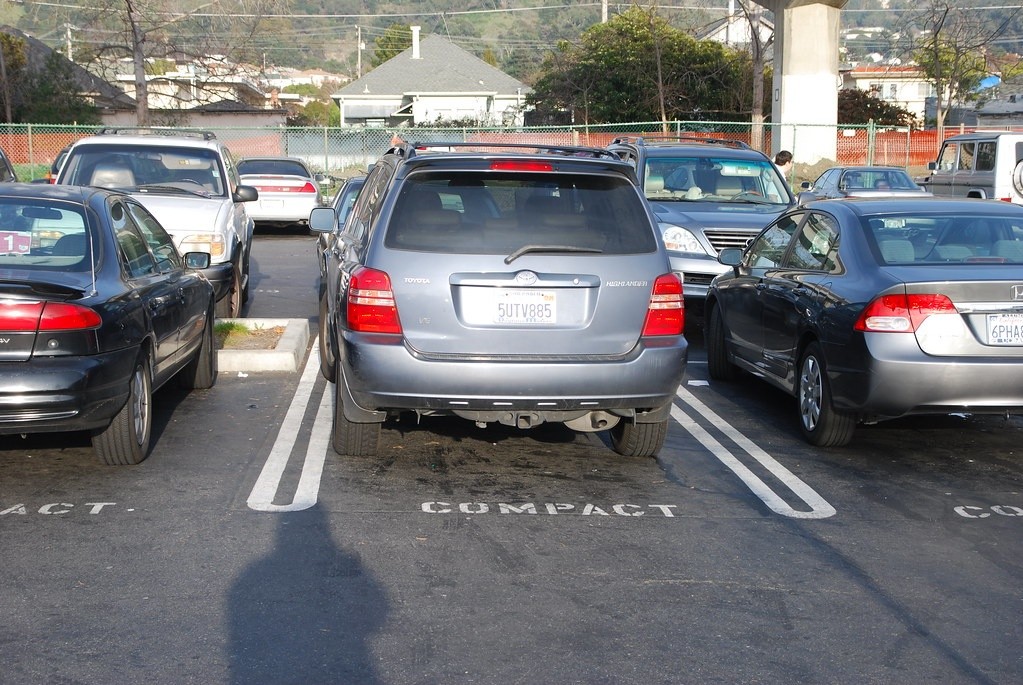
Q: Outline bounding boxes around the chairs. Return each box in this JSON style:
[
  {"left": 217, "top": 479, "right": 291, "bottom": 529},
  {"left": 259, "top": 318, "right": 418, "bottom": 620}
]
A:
[
  {"left": 644, "top": 176, "right": 666, "bottom": 197},
  {"left": 51, "top": 233, "right": 87, "bottom": 257},
  {"left": 527, "top": 196, "right": 560, "bottom": 221},
  {"left": 408, "top": 189, "right": 444, "bottom": 209},
  {"left": 875, "top": 179, "right": 889, "bottom": 188},
  {"left": 716, "top": 176, "right": 744, "bottom": 198},
  {"left": 89, "top": 164, "right": 135, "bottom": 192}
]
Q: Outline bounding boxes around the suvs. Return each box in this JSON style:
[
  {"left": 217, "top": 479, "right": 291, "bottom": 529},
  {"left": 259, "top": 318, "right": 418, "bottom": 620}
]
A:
[
  {"left": 47, "top": 124, "right": 259, "bottom": 329},
  {"left": 918, "top": 130, "right": 1023, "bottom": 210},
  {"left": 598, "top": 134, "right": 800, "bottom": 326},
  {"left": 41, "top": 142, "right": 169, "bottom": 191},
  {"left": 309, "top": 140, "right": 688, "bottom": 461}
]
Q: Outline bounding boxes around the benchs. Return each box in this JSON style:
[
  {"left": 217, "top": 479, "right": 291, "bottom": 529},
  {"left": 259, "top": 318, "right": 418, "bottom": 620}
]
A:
[
  {"left": 879, "top": 240, "right": 1023, "bottom": 264},
  {"left": 396, "top": 209, "right": 607, "bottom": 251}
]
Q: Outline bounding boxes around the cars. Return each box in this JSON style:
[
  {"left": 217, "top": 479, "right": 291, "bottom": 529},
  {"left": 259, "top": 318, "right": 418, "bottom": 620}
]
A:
[
  {"left": 0, "top": 146, "right": 19, "bottom": 186},
  {"left": 233, "top": 157, "right": 330, "bottom": 234},
  {"left": 704, "top": 195, "right": 1023, "bottom": 443},
  {"left": 316, "top": 178, "right": 505, "bottom": 282},
  {"left": 0, "top": 180, "right": 218, "bottom": 465},
  {"left": 789, "top": 164, "right": 946, "bottom": 232}
]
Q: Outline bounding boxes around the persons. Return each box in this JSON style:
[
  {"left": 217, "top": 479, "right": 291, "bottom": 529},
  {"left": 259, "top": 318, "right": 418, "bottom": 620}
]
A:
[{"left": 763, "top": 150, "right": 793, "bottom": 203}]
